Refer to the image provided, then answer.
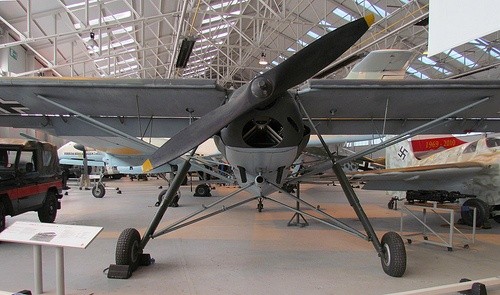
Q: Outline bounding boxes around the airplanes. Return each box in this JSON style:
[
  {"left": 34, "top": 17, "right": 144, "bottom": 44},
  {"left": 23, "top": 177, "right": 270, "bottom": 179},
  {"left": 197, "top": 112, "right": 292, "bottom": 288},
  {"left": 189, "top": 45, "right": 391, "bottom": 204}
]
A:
[
  {"left": 301, "top": 134, "right": 500, "bottom": 228},
  {"left": 0, "top": 14, "right": 500, "bottom": 277},
  {"left": 57, "top": 137, "right": 226, "bottom": 197}
]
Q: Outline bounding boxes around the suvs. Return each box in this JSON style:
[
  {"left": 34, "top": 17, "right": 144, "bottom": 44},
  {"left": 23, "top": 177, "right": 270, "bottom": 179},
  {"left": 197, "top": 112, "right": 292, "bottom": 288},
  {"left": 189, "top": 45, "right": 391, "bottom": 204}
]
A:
[{"left": 0, "top": 137, "right": 63, "bottom": 231}]
